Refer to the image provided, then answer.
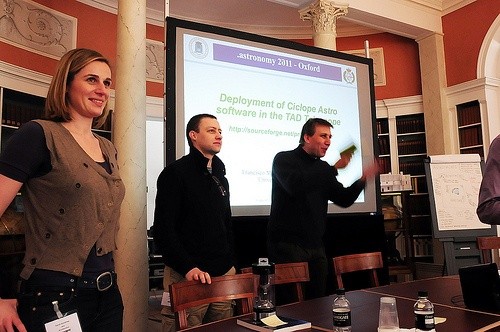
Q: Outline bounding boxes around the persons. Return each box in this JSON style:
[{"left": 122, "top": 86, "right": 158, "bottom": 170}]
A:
[
  {"left": 153, "top": 113, "right": 235, "bottom": 332},
  {"left": 0, "top": 48, "right": 125, "bottom": 332},
  {"left": 272, "top": 118, "right": 369, "bottom": 306},
  {"left": 476, "top": 134, "right": 500, "bottom": 225}
]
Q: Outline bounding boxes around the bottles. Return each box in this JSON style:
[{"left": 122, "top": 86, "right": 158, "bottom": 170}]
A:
[
  {"left": 413, "top": 290, "right": 436, "bottom": 332},
  {"left": 332, "top": 288, "right": 352, "bottom": 332},
  {"left": 252, "top": 261, "right": 275, "bottom": 325}
]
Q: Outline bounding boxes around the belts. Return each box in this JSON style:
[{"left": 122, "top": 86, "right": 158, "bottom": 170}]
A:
[{"left": 34, "top": 268, "right": 118, "bottom": 292}]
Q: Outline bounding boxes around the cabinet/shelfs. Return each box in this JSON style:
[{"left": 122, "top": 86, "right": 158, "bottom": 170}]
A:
[{"left": 376, "top": 99, "right": 486, "bottom": 264}]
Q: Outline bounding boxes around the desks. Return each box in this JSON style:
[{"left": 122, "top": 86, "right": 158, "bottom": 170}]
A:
[
  {"left": 356, "top": 274, "right": 500, "bottom": 316},
  {"left": 177, "top": 290, "right": 500, "bottom": 332}
]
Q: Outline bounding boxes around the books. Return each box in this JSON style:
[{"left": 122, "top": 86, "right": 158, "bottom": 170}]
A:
[{"left": 237, "top": 315, "right": 311, "bottom": 332}]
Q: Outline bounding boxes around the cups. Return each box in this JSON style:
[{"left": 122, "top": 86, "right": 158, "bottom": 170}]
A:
[{"left": 377, "top": 296, "right": 399, "bottom": 332}]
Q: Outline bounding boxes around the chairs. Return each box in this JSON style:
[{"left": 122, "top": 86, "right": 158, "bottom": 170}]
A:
[
  {"left": 477, "top": 237, "right": 500, "bottom": 264},
  {"left": 169, "top": 272, "right": 260, "bottom": 331},
  {"left": 333, "top": 252, "right": 384, "bottom": 290},
  {"left": 240, "top": 262, "right": 310, "bottom": 313}
]
